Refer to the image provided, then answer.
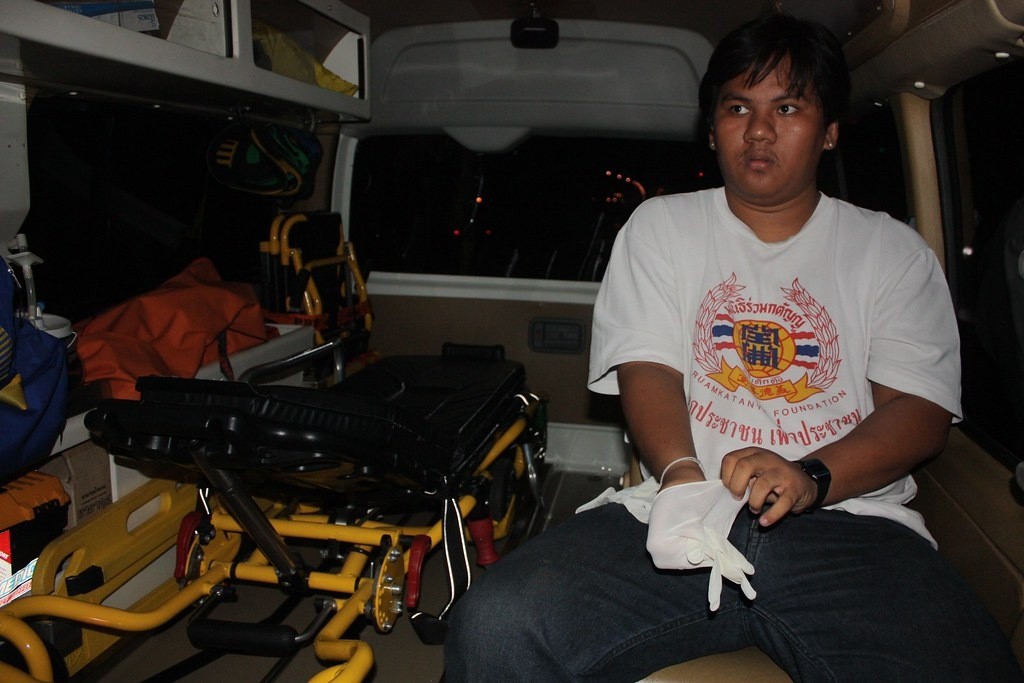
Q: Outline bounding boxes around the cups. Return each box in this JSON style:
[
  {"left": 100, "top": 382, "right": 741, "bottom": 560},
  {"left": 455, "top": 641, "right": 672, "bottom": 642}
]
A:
[{"left": 37, "top": 313, "right": 71, "bottom": 337}]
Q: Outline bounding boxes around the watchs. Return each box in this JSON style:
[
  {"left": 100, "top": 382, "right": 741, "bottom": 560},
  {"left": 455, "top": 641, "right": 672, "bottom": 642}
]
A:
[{"left": 792, "top": 455, "right": 832, "bottom": 511}]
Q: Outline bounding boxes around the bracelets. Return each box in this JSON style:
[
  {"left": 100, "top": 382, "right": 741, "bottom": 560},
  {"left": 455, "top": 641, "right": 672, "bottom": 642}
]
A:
[{"left": 658, "top": 456, "right": 706, "bottom": 488}]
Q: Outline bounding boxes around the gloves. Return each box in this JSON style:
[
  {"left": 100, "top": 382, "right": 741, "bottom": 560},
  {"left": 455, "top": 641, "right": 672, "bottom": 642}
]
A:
[
  {"left": 574, "top": 474, "right": 662, "bottom": 524},
  {"left": 646, "top": 478, "right": 756, "bottom": 611}
]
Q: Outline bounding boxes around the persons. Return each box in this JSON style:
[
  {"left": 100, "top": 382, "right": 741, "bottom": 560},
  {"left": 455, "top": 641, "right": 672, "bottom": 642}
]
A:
[{"left": 447, "top": 13, "right": 1024, "bottom": 683}]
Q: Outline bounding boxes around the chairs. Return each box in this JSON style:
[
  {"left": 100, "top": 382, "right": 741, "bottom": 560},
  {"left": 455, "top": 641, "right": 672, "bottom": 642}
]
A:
[{"left": 84, "top": 354, "right": 529, "bottom": 530}]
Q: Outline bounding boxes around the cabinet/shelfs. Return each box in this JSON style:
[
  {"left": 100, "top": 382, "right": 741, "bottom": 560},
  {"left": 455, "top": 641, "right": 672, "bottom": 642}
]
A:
[{"left": 0, "top": 0, "right": 372, "bottom": 125}]
